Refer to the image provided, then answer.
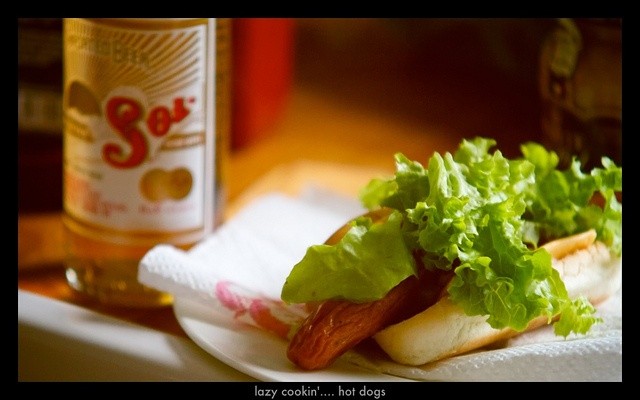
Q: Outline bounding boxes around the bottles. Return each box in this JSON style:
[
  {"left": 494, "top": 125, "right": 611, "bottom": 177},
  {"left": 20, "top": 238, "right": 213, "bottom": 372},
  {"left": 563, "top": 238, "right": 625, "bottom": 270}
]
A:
[{"left": 58, "top": 16, "right": 233, "bottom": 310}]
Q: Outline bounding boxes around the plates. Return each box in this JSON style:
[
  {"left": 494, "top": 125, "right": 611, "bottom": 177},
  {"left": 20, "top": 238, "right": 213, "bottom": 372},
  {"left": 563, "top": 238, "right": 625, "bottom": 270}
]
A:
[{"left": 171, "top": 189, "right": 419, "bottom": 382}]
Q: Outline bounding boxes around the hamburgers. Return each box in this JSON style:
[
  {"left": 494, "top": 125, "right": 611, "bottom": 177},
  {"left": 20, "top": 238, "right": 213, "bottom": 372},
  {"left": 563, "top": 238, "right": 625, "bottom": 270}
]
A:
[{"left": 281, "top": 134, "right": 623, "bottom": 371}]
